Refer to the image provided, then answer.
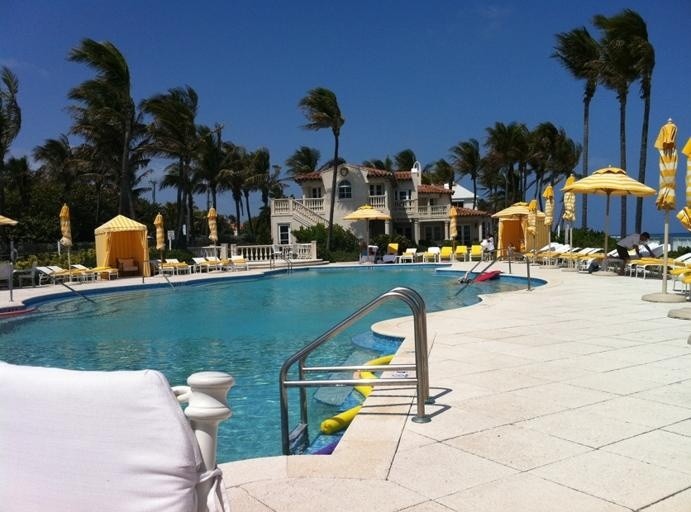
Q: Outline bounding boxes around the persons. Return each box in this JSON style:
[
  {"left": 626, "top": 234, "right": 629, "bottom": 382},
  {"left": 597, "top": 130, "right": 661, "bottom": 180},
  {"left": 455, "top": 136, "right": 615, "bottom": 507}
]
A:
[
  {"left": 480, "top": 233, "right": 495, "bottom": 261},
  {"left": 615, "top": 231, "right": 655, "bottom": 276},
  {"left": 507, "top": 242, "right": 513, "bottom": 256}
]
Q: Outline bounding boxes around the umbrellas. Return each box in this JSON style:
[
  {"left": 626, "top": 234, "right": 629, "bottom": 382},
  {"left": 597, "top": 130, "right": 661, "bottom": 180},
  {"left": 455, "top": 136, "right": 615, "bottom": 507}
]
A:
[
  {"left": 527, "top": 199, "right": 538, "bottom": 264},
  {"left": 676, "top": 136, "right": 691, "bottom": 234},
  {"left": 59, "top": 203, "right": 73, "bottom": 283},
  {"left": 654, "top": 117, "right": 678, "bottom": 294},
  {"left": 560, "top": 164, "right": 658, "bottom": 272},
  {"left": 153, "top": 212, "right": 166, "bottom": 269},
  {"left": 342, "top": 202, "right": 392, "bottom": 261},
  {"left": 542, "top": 184, "right": 554, "bottom": 265},
  {"left": 207, "top": 208, "right": 219, "bottom": 270},
  {"left": 448, "top": 205, "right": 458, "bottom": 261},
  {"left": 562, "top": 173, "right": 576, "bottom": 268},
  {"left": 0, "top": 214, "right": 18, "bottom": 226}
]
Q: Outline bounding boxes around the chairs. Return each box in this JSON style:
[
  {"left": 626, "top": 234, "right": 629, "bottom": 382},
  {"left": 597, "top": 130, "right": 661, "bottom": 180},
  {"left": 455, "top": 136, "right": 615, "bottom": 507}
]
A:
[
  {"left": 14, "top": 254, "right": 250, "bottom": 293},
  {"left": 525, "top": 242, "right": 670, "bottom": 280},
  {"left": 360, "top": 242, "right": 484, "bottom": 263},
  {"left": 628, "top": 253, "right": 691, "bottom": 323}
]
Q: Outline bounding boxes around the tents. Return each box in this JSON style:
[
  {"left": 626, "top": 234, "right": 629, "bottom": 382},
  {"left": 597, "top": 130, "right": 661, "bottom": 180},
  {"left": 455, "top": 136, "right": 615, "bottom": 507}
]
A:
[{"left": 491, "top": 200, "right": 550, "bottom": 262}]
